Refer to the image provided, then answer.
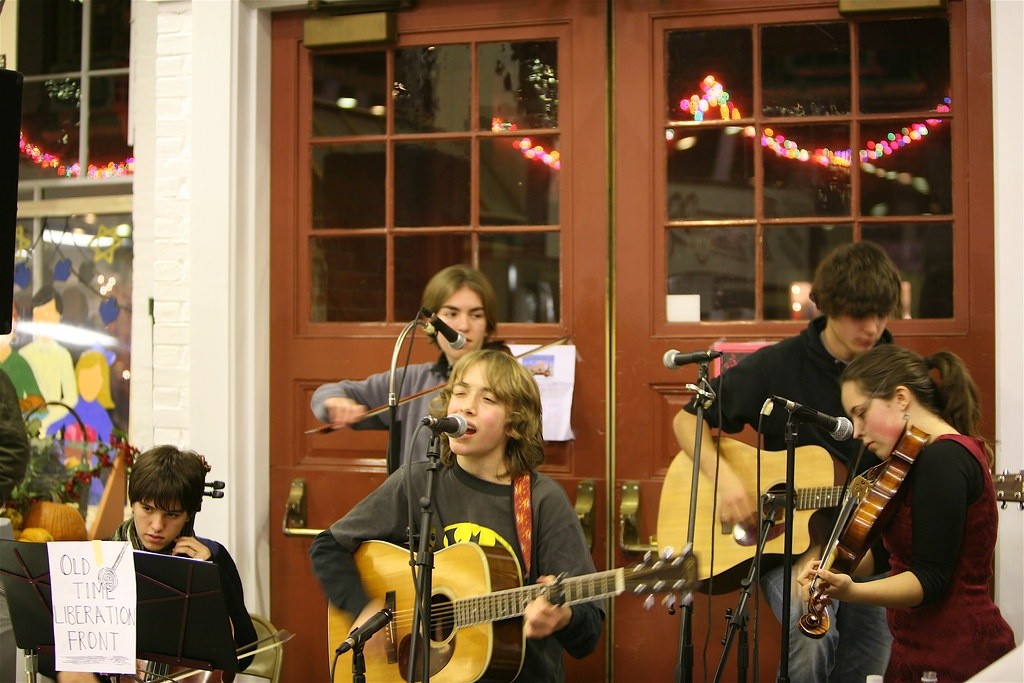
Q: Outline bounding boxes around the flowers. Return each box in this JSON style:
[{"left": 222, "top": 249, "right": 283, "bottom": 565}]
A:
[{"left": 0, "top": 417, "right": 142, "bottom": 517}]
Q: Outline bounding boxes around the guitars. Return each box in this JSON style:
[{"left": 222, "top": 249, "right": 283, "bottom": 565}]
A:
[
  {"left": 327, "top": 540, "right": 699, "bottom": 683},
  {"left": 657, "top": 435, "right": 1023, "bottom": 597}
]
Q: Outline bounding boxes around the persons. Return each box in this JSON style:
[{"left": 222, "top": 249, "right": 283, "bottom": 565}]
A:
[
  {"left": 796, "top": 344, "right": 1016, "bottom": 683},
  {"left": 0, "top": 365, "right": 30, "bottom": 507},
  {"left": 308, "top": 349, "right": 606, "bottom": 683},
  {"left": 311, "top": 265, "right": 499, "bottom": 468},
  {"left": 673, "top": 241, "right": 902, "bottom": 683},
  {"left": 38, "top": 445, "right": 257, "bottom": 683}
]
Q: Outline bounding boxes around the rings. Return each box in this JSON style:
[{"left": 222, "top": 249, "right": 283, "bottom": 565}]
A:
[{"left": 193, "top": 551, "right": 196, "bottom": 558}]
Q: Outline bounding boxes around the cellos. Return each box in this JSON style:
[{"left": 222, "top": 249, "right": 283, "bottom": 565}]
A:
[{"left": 115, "top": 454, "right": 226, "bottom": 683}]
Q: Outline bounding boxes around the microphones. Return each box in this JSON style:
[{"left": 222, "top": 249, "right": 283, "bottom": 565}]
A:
[
  {"left": 422, "top": 309, "right": 467, "bottom": 350},
  {"left": 770, "top": 395, "right": 854, "bottom": 441},
  {"left": 762, "top": 492, "right": 797, "bottom": 509},
  {"left": 662, "top": 349, "right": 722, "bottom": 370},
  {"left": 420, "top": 412, "right": 468, "bottom": 439},
  {"left": 336, "top": 608, "right": 394, "bottom": 654}
]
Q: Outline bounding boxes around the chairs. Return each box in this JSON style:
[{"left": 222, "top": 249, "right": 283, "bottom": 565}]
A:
[{"left": 229, "top": 614, "right": 285, "bottom": 683}]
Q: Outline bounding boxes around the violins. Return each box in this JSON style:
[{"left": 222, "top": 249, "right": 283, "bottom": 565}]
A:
[
  {"left": 796, "top": 425, "right": 931, "bottom": 639},
  {"left": 483, "top": 340, "right": 551, "bottom": 377}
]
